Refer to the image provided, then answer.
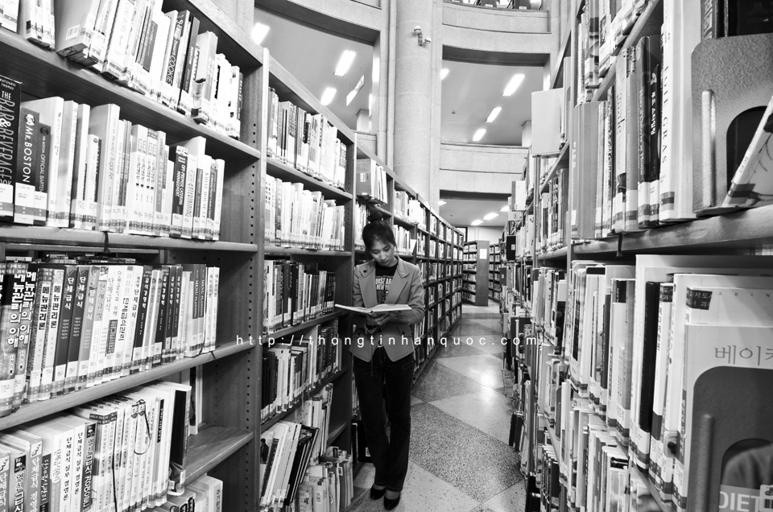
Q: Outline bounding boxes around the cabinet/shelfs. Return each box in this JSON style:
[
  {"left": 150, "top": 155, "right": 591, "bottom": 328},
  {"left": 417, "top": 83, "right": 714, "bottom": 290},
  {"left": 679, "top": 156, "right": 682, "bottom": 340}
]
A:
[
  {"left": 0, "top": 0, "right": 267, "bottom": 511},
  {"left": 351, "top": 137, "right": 464, "bottom": 477},
  {"left": 465, "top": 241, "right": 490, "bottom": 307},
  {"left": 259, "top": 48, "right": 355, "bottom": 512}
]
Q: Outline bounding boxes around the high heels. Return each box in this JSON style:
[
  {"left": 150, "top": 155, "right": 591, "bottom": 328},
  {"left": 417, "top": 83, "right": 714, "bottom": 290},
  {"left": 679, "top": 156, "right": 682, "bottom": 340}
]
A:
[
  {"left": 383, "top": 490, "right": 400, "bottom": 511},
  {"left": 369, "top": 483, "right": 386, "bottom": 500}
]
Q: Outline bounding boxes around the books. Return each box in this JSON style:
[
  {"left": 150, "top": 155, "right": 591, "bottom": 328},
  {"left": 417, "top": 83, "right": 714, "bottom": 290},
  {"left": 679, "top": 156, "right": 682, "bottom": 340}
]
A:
[{"left": 0, "top": 0, "right": 773, "bottom": 512}]
